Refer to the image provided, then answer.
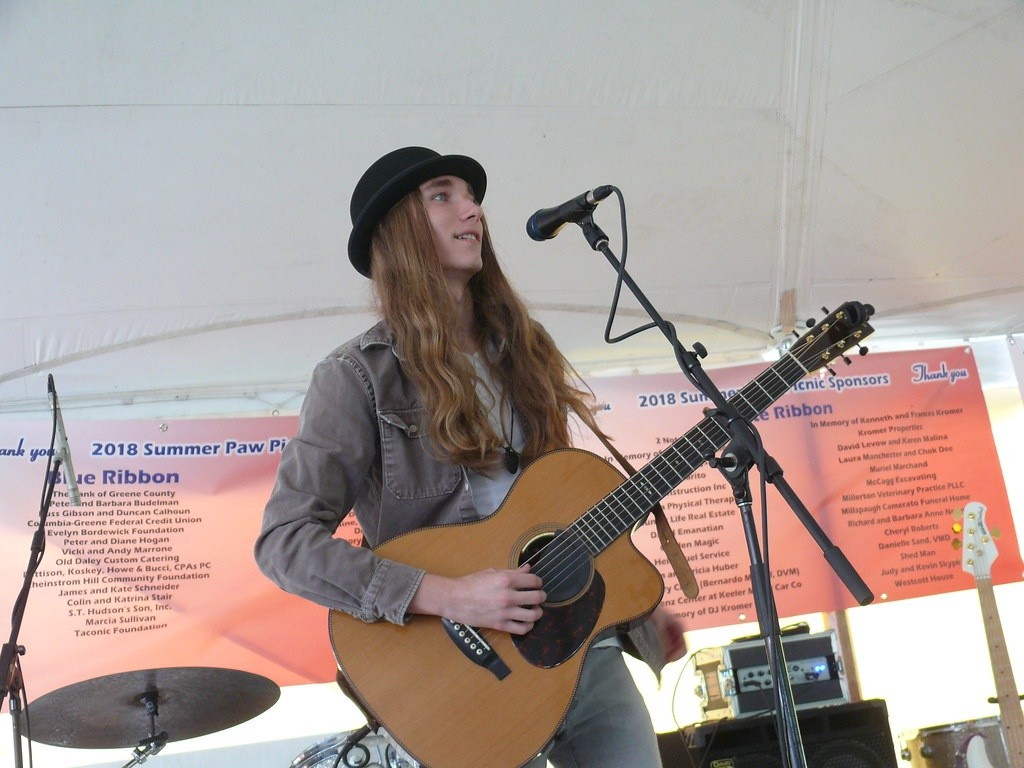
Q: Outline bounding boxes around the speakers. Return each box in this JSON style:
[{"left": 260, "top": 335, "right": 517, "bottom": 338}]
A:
[{"left": 657, "top": 698, "right": 898, "bottom": 768}]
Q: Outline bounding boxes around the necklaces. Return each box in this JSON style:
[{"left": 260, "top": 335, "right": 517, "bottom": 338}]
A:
[{"left": 500, "top": 406, "right": 518, "bottom": 475}]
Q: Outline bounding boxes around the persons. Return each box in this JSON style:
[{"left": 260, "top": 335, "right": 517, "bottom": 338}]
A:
[{"left": 254, "top": 146, "right": 687, "bottom": 768}]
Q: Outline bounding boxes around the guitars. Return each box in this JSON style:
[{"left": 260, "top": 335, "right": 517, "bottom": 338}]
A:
[
  {"left": 952, "top": 500, "right": 1024, "bottom": 768},
  {"left": 324, "top": 299, "right": 876, "bottom": 768}
]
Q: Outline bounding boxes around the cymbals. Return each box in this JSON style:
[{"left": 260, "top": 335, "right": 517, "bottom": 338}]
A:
[{"left": 21, "top": 666, "right": 280, "bottom": 749}]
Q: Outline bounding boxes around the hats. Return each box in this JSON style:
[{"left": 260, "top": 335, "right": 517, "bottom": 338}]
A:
[{"left": 348, "top": 146, "right": 487, "bottom": 280}]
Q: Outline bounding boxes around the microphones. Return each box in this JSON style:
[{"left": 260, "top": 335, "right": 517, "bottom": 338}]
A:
[
  {"left": 526, "top": 185, "right": 614, "bottom": 241},
  {"left": 47, "top": 381, "right": 81, "bottom": 506}
]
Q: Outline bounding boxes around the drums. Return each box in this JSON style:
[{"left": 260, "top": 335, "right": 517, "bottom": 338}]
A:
[{"left": 898, "top": 714, "right": 1009, "bottom": 767}]
